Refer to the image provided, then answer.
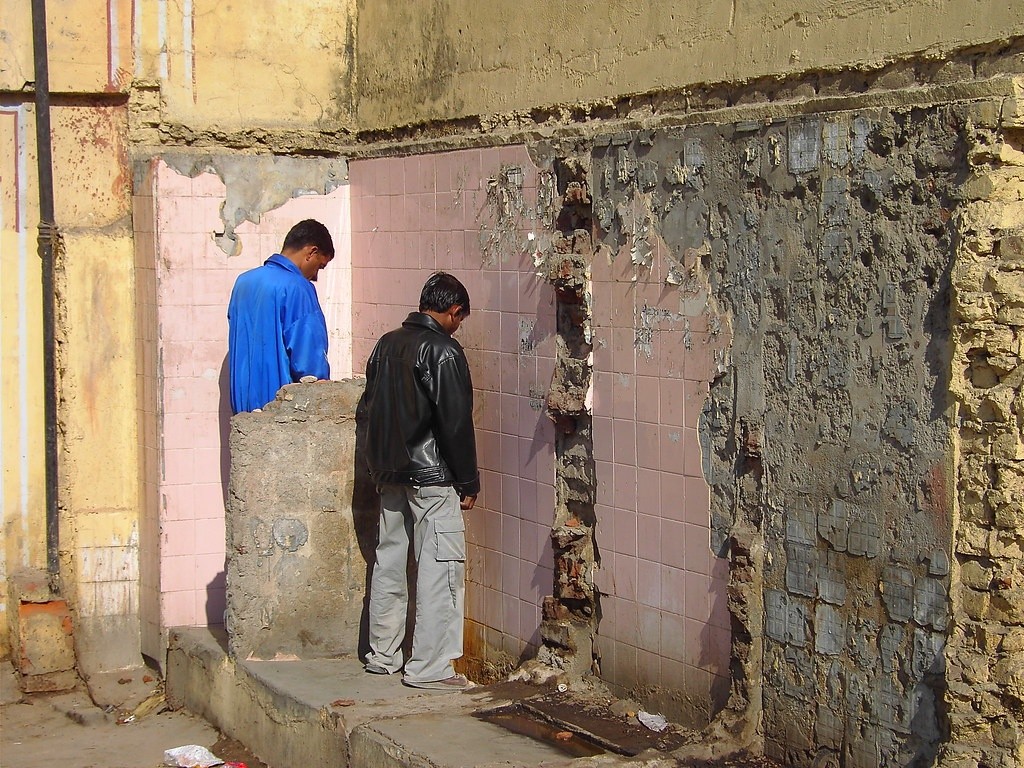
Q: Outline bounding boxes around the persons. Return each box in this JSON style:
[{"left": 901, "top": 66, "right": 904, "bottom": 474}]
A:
[
  {"left": 228, "top": 219, "right": 335, "bottom": 415},
  {"left": 364, "top": 268, "right": 480, "bottom": 690}
]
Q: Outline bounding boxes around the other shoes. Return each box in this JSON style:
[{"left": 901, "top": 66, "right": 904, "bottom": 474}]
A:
[
  {"left": 364, "top": 662, "right": 387, "bottom": 674},
  {"left": 403, "top": 672, "right": 475, "bottom": 690}
]
